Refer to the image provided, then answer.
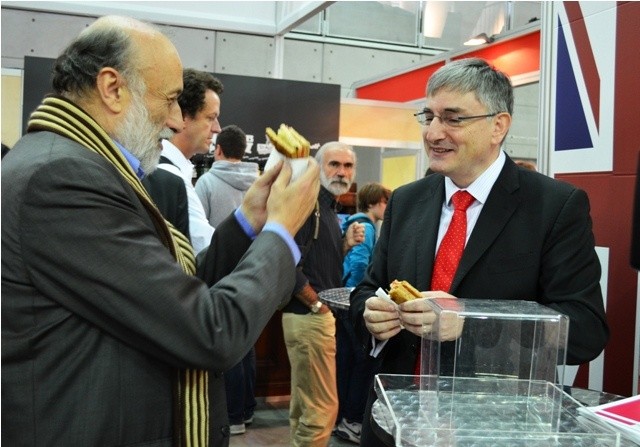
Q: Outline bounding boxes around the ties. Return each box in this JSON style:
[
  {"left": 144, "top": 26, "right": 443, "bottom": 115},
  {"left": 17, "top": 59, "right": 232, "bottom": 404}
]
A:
[{"left": 415, "top": 191, "right": 475, "bottom": 387}]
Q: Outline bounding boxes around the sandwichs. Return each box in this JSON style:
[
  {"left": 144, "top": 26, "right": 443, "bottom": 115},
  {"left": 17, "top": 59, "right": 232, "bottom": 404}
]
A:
[
  {"left": 388, "top": 279, "right": 425, "bottom": 305},
  {"left": 263, "top": 123, "right": 310, "bottom": 159}
]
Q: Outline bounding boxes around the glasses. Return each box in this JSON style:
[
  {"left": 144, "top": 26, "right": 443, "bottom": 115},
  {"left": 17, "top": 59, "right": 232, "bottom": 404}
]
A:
[{"left": 413, "top": 110, "right": 493, "bottom": 130}]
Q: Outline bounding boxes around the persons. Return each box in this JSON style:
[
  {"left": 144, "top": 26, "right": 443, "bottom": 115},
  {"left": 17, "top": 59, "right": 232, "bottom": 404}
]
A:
[
  {"left": 337, "top": 182, "right": 392, "bottom": 444},
  {"left": 282, "top": 139, "right": 358, "bottom": 447},
  {"left": 348, "top": 55, "right": 611, "bottom": 446},
  {"left": 194, "top": 124, "right": 263, "bottom": 436},
  {"left": 155, "top": 66, "right": 217, "bottom": 261},
  {"left": 1, "top": 11, "right": 321, "bottom": 445}
]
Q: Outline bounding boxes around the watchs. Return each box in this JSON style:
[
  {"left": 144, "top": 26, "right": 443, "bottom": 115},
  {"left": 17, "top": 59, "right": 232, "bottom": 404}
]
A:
[{"left": 309, "top": 299, "right": 322, "bottom": 316}]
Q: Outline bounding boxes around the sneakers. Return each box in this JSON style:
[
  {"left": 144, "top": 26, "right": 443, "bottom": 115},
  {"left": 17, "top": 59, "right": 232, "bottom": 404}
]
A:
[
  {"left": 337, "top": 417, "right": 363, "bottom": 444},
  {"left": 244, "top": 410, "right": 256, "bottom": 423},
  {"left": 230, "top": 423, "right": 246, "bottom": 434}
]
